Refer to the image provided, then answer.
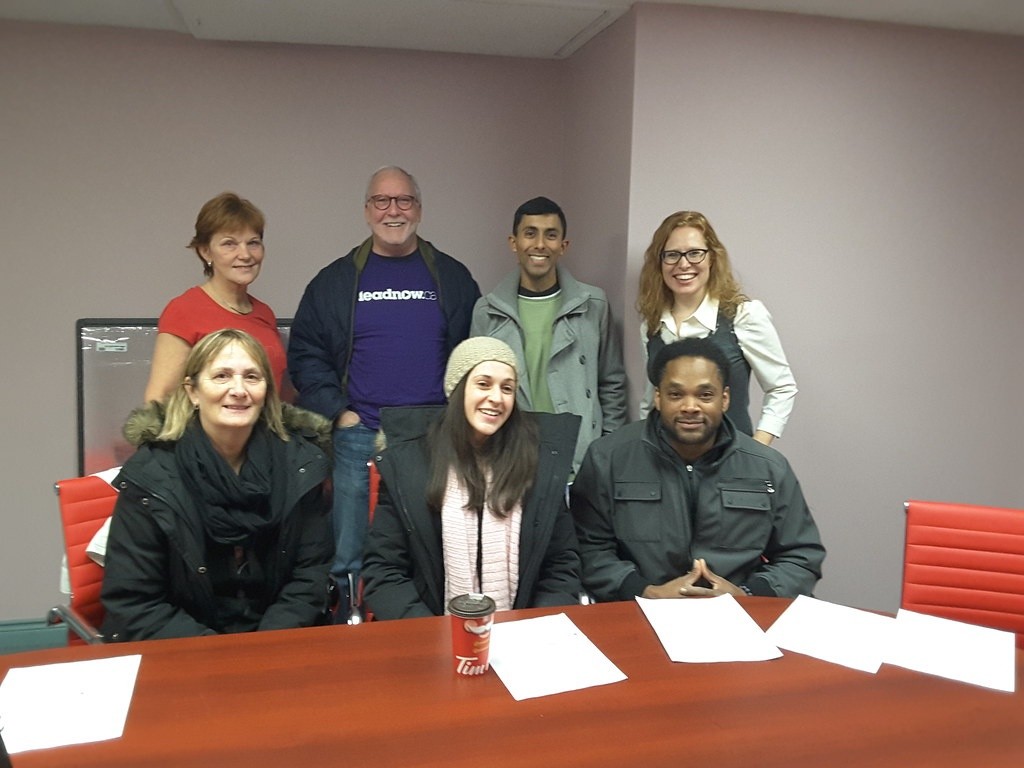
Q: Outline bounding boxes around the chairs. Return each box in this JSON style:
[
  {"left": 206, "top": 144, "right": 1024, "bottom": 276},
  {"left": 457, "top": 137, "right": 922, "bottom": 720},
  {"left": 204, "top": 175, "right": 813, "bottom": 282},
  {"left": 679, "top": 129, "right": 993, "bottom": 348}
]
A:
[
  {"left": 47, "top": 465, "right": 122, "bottom": 647},
  {"left": 347, "top": 459, "right": 382, "bottom": 625},
  {"left": 899, "top": 499, "right": 1024, "bottom": 648}
]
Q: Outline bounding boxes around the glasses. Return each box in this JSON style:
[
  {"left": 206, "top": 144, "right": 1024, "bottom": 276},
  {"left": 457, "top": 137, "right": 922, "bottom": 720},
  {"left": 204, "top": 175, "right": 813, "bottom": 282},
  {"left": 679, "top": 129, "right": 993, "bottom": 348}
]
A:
[
  {"left": 660, "top": 249, "right": 708, "bottom": 265},
  {"left": 365, "top": 193, "right": 422, "bottom": 211}
]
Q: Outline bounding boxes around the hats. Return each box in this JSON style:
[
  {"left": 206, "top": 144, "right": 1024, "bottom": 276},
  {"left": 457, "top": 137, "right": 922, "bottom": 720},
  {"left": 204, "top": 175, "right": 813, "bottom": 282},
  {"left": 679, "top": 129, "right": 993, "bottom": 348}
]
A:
[{"left": 442, "top": 336, "right": 520, "bottom": 398}]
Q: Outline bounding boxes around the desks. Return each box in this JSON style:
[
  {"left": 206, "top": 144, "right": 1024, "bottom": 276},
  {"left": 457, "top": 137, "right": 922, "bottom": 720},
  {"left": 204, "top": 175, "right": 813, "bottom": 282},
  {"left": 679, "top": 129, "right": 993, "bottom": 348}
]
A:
[{"left": 1, "top": 594, "right": 1024, "bottom": 768}]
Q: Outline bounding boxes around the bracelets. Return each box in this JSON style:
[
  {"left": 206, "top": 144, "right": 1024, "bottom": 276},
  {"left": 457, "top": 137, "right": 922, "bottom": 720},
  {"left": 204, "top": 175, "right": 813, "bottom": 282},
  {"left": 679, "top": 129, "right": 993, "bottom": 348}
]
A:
[{"left": 738, "top": 586, "right": 754, "bottom": 596}]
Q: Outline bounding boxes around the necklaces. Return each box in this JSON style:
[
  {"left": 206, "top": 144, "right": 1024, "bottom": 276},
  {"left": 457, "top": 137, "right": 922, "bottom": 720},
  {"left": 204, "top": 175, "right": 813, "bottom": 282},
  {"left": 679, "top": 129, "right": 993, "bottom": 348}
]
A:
[{"left": 206, "top": 282, "right": 253, "bottom": 314}]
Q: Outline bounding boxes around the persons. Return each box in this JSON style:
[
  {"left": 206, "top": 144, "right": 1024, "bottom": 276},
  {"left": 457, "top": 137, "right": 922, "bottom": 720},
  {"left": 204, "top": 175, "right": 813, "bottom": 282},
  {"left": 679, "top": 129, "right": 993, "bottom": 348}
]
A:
[
  {"left": 287, "top": 167, "right": 483, "bottom": 626},
  {"left": 100, "top": 329, "right": 335, "bottom": 640},
  {"left": 470, "top": 197, "right": 629, "bottom": 502},
  {"left": 144, "top": 193, "right": 295, "bottom": 409},
  {"left": 363, "top": 337, "right": 585, "bottom": 618},
  {"left": 569, "top": 337, "right": 827, "bottom": 604},
  {"left": 639, "top": 210, "right": 799, "bottom": 446}
]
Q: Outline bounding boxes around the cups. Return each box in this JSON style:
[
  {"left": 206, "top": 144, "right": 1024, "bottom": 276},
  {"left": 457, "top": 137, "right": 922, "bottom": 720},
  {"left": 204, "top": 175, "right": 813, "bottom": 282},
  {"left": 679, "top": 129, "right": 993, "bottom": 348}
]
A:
[{"left": 446, "top": 593, "right": 496, "bottom": 678}]
